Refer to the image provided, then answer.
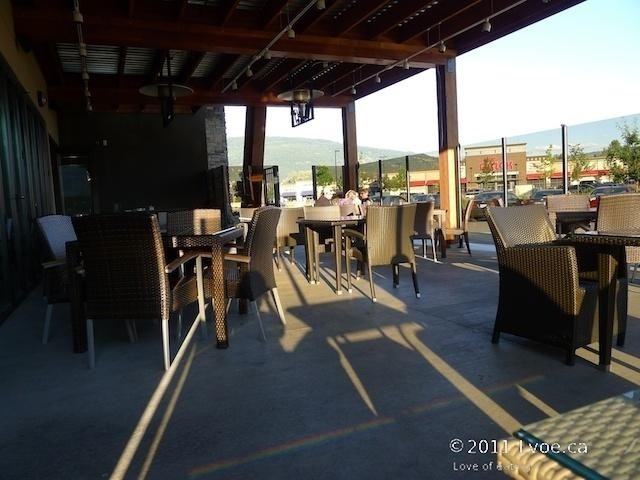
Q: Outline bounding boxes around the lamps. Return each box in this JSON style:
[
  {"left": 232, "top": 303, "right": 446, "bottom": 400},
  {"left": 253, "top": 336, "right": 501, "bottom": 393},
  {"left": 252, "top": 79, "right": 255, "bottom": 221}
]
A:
[
  {"left": 277, "top": 74, "right": 325, "bottom": 127},
  {"left": 332, "top": 0, "right": 531, "bottom": 99},
  {"left": 138, "top": 54, "right": 195, "bottom": 129},
  {"left": 221, "top": 0, "right": 325, "bottom": 95},
  {"left": 72, "top": 0, "right": 94, "bottom": 113}
]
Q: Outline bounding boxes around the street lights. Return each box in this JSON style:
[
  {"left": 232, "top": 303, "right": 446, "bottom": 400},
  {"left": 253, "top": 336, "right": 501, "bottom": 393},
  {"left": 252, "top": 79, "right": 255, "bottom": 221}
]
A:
[{"left": 332, "top": 149, "right": 341, "bottom": 185}]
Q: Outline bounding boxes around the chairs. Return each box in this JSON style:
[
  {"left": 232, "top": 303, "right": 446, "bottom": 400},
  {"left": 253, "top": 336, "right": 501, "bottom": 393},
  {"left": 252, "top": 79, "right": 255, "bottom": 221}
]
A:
[{"left": 35, "top": 194, "right": 640, "bottom": 371}]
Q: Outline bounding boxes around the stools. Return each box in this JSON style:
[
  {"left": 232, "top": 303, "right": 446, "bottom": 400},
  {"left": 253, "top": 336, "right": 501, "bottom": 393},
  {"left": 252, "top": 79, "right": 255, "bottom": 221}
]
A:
[{"left": 497, "top": 388, "right": 640, "bottom": 480}]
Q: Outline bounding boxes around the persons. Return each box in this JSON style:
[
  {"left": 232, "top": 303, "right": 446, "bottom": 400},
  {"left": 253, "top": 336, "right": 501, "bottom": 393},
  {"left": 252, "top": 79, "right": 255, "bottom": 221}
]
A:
[
  {"left": 359, "top": 189, "right": 373, "bottom": 205},
  {"left": 316, "top": 186, "right": 336, "bottom": 207},
  {"left": 343, "top": 190, "right": 361, "bottom": 205}
]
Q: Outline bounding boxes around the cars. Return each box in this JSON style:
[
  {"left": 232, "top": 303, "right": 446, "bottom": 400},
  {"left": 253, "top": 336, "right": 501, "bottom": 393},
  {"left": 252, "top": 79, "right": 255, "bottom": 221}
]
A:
[{"left": 361, "top": 179, "right": 635, "bottom": 224}]
